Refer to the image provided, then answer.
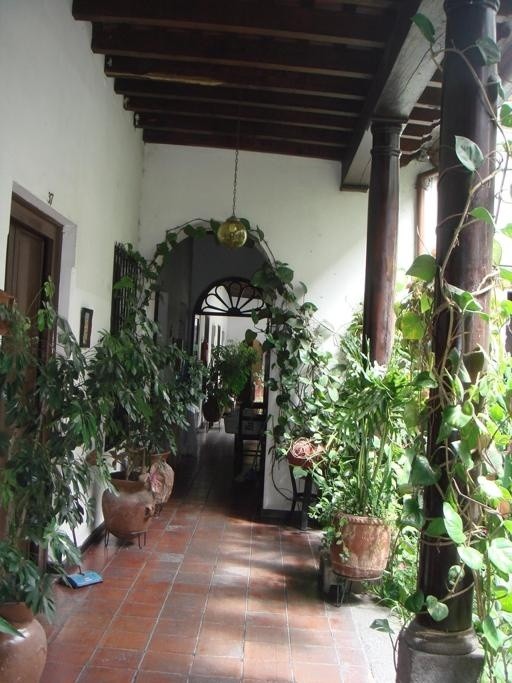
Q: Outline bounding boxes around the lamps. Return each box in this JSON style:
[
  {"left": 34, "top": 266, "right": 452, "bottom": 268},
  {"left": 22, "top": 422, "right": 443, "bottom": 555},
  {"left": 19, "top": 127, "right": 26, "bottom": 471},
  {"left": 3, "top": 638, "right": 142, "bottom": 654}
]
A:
[{"left": 215, "top": 148, "right": 248, "bottom": 251}]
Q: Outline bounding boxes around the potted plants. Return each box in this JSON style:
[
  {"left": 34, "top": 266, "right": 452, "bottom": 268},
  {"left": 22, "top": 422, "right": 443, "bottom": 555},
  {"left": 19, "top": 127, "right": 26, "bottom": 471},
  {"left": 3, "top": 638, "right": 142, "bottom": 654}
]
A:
[
  {"left": 258, "top": 267, "right": 439, "bottom": 605},
  {"left": 1, "top": 235, "right": 257, "bottom": 683}
]
